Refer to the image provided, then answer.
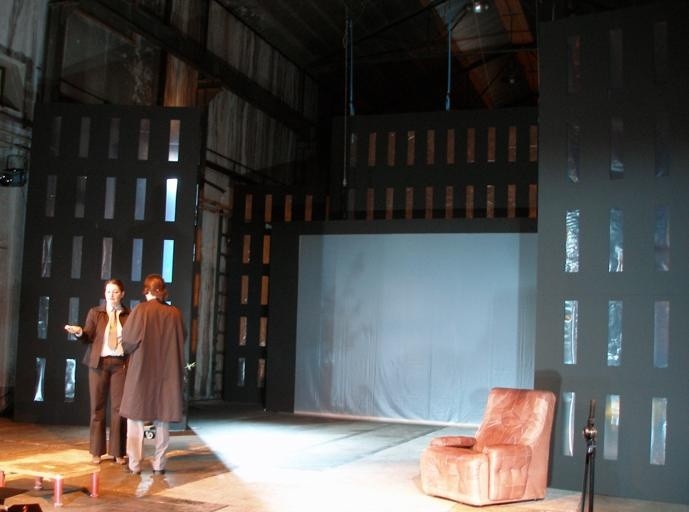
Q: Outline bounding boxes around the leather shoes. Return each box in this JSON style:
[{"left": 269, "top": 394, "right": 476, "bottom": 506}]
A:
[{"left": 91, "top": 455, "right": 126, "bottom": 465}]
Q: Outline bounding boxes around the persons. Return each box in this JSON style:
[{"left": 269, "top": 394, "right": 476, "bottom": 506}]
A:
[
  {"left": 63, "top": 278, "right": 132, "bottom": 466},
  {"left": 118, "top": 274, "right": 185, "bottom": 476}
]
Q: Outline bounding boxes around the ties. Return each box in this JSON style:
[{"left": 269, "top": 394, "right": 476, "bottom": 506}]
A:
[{"left": 108, "top": 309, "right": 118, "bottom": 351}]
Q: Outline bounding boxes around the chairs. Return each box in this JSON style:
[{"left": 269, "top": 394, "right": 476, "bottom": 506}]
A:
[{"left": 421, "top": 388, "right": 557, "bottom": 506}]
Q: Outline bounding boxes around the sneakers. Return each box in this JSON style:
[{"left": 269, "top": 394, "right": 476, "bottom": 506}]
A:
[{"left": 130, "top": 470, "right": 166, "bottom": 475}]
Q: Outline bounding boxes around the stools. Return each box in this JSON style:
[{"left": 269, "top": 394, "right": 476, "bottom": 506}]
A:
[{"left": 0, "top": 460, "right": 100, "bottom": 507}]
